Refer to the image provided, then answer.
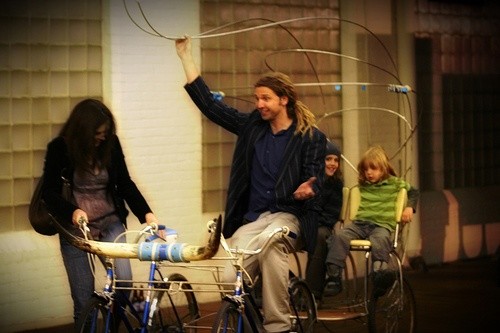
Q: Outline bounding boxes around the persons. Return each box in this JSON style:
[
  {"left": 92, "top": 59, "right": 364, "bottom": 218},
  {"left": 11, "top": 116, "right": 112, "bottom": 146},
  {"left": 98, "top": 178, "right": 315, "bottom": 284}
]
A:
[
  {"left": 43, "top": 98, "right": 165, "bottom": 333},
  {"left": 174, "top": 32, "right": 328, "bottom": 333},
  {"left": 302, "top": 140, "right": 345, "bottom": 309},
  {"left": 324, "top": 143, "right": 420, "bottom": 299}
]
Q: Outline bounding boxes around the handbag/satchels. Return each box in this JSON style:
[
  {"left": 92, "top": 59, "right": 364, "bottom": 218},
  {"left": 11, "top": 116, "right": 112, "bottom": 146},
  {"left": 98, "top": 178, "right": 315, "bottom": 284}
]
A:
[{"left": 29, "top": 173, "right": 60, "bottom": 236}]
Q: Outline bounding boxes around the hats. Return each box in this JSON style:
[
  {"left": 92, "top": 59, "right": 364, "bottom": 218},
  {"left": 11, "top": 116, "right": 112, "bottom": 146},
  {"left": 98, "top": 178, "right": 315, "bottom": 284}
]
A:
[{"left": 325, "top": 142, "right": 340, "bottom": 155}]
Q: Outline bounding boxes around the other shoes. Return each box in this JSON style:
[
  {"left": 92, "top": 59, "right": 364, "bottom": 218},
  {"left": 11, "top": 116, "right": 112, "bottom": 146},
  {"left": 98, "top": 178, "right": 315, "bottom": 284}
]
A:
[
  {"left": 325, "top": 273, "right": 341, "bottom": 296},
  {"left": 371, "top": 269, "right": 396, "bottom": 296}
]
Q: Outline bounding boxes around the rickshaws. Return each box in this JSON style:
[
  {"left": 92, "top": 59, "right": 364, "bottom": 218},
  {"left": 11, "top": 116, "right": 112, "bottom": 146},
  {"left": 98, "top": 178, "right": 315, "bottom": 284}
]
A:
[{"left": 44, "top": 0, "right": 425, "bottom": 333}]
